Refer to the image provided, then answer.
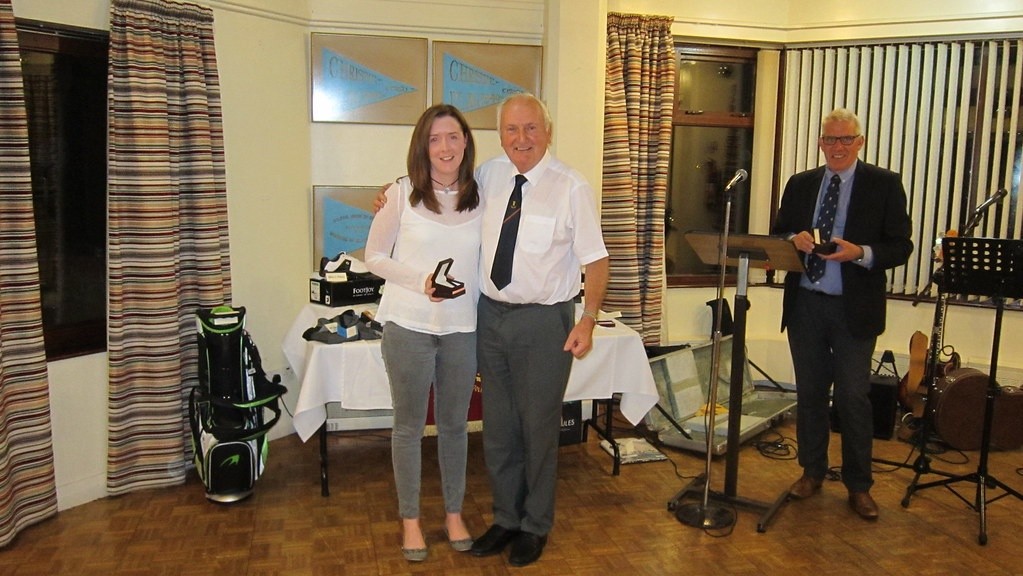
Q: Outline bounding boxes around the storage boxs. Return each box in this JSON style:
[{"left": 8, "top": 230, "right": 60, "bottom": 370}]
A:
[{"left": 558, "top": 398, "right": 582, "bottom": 447}]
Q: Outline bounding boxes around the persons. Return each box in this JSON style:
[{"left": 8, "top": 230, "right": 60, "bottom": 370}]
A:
[
  {"left": 770, "top": 108, "right": 914, "bottom": 516},
  {"left": 373, "top": 94, "right": 609, "bottom": 567},
  {"left": 365, "top": 103, "right": 485, "bottom": 562}
]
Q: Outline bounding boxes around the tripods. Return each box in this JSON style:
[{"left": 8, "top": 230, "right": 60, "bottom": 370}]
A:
[{"left": 871, "top": 209, "right": 1023, "bottom": 546}]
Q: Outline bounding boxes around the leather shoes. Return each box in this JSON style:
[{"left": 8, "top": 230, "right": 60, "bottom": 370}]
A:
[
  {"left": 509, "top": 529, "right": 548, "bottom": 566},
  {"left": 469, "top": 525, "right": 519, "bottom": 557}
]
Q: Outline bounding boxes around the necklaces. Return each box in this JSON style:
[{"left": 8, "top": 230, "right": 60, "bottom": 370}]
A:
[{"left": 431, "top": 177, "right": 459, "bottom": 193}]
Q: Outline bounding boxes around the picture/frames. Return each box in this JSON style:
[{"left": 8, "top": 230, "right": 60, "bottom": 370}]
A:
[
  {"left": 432, "top": 40, "right": 544, "bottom": 129},
  {"left": 311, "top": 32, "right": 428, "bottom": 126},
  {"left": 312, "top": 185, "right": 383, "bottom": 272}
]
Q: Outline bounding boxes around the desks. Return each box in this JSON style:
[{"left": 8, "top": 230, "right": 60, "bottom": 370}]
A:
[
  {"left": 310, "top": 274, "right": 384, "bottom": 307},
  {"left": 283, "top": 302, "right": 660, "bottom": 496}
]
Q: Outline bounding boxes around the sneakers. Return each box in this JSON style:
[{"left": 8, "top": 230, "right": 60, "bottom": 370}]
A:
[
  {"left": 848, "top": 491, "right": 879, "bottom": 520},
  {"left": 789, "top": 473, "right": 822, "bottom": 498}
]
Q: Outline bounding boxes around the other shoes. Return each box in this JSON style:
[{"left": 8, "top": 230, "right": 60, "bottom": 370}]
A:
[
  {"left": 442, "top": 520, "right": 472, "bottom": 551},
  {"left": 400, "top": 530, "right": 426, "bottom": 561}
]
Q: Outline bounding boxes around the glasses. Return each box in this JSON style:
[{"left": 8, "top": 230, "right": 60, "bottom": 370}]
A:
[{"left": 821, "top": 133, "right": 860, "bottom": 146}]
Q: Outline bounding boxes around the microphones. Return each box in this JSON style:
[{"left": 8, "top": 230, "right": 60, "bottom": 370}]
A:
[
  {"left": 725, "top": 169, "right": 748, "bottom": 192},
  {"left": 974, "top": 189, "right": 1008, "bottom": 214}
]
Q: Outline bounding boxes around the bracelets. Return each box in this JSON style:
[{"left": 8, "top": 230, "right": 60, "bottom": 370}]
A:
[
  {"left": 857, "top": 259, "right": 863, "bottom": 262},
  {"left": 582, "top": 311, "right": 599, "bottom": 324}
]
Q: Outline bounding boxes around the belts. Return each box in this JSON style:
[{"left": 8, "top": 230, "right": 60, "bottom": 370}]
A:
[{"left": 490, "top": 299, "right": 520, "bottom": 308}]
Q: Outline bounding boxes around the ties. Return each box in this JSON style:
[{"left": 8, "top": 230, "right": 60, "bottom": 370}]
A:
[
  {"left": 803, "top": 173, "right": 841, "bottom": 284},
  {"left": 490, "top": 174, "right": 528, "bottom": 293}
]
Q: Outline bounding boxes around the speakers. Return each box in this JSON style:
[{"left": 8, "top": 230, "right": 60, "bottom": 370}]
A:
[{"left": 831, "top": 373, "right": 899, "bottom": 440}]
